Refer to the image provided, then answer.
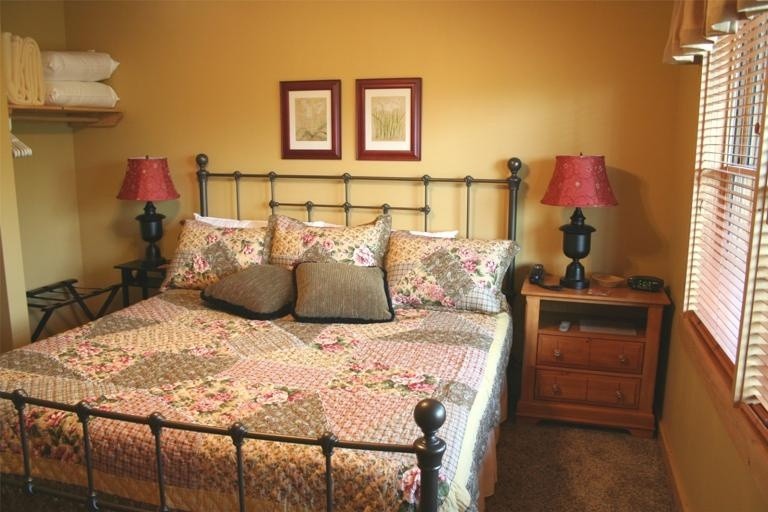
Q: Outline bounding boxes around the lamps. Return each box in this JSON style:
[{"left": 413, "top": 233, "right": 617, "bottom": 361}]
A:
[
  {"left": 115, "top": 155, "right": 181, "bottom": 267},
  {"left": 540, "top": 153, "right": 621, "bottom": 290}
]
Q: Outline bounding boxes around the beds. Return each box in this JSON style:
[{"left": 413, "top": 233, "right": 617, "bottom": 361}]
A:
[{"left": 0, "top": 154, "right": 525, "bottom": 512}]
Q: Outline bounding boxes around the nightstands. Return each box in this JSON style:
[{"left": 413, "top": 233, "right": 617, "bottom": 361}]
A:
[{"left": 519, "top": 273, "right": 672, "bottom": 438}]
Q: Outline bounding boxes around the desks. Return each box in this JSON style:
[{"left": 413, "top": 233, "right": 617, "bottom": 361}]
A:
[{"left": 114, "top": 259, "right": 170, "bottom": 308}]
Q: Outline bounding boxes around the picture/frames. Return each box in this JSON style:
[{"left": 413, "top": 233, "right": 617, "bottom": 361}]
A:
[
  {"left": 280, "top": 81, "right": 341, "bottom": 159},
  {"left": 355, "top": 79, "right": 421, "bottom": 161}
]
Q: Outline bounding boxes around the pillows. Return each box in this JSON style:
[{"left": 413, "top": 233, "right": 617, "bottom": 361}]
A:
[
  {"left": 386, "top": 229, "right": 520, "bottom": 314},
  {"left": 45, "top": 82, "right": 120, "bottom": 108},
  {"left": 266, "top": 214, "right": 393, "bottom": 266},
  {"left": 42, "top": 50, "right": 120, "bottom": 81},
  {"left": 292, "top": 260, "right": 393, "bottom": 322},
  {"left": 199, "top": 263, "right": 295, "bottom": 320},
  {"left": 161, "top": 215, "right": 274, "bottom": 287}
]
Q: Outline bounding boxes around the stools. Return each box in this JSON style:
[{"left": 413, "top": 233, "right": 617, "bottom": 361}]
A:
[{"left": 27, "top": 278, "right": 121, "bottom": 343}]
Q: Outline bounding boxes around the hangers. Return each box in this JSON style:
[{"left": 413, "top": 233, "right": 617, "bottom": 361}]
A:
[{"left": 7, "top": 118, "right": 32, "bottom": 157}]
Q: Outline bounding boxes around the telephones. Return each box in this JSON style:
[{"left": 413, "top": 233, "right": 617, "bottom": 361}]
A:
[{"left": 529, "top": 264, "right": 544, "bottom": 284}]
